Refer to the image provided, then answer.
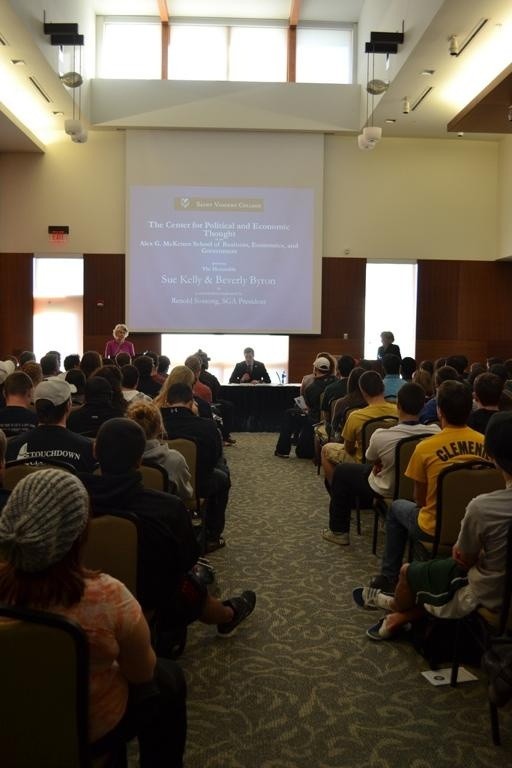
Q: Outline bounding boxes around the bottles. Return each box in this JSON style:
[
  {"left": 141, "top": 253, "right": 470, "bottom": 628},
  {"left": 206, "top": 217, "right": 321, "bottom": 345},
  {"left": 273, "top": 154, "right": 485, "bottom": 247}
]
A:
[{"left": 282, "top": 370, "right": 287, "bottom": 384}]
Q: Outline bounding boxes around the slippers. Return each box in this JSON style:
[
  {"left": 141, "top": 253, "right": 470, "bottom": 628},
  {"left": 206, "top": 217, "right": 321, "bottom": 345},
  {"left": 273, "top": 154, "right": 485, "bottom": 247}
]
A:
[{"left": 350, "top": 582, "right": 395, "bottom": 642}]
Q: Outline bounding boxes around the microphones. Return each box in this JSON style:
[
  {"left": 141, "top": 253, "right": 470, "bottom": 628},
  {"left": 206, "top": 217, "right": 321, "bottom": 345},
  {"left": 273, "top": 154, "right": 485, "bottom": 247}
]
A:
[{"left": 275, "top": 371, "right": 283, "bottom": 385}]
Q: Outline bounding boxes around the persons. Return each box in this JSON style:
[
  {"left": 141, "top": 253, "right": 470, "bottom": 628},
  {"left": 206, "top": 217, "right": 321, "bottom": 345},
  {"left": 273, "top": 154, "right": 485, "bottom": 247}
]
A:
[
  {"left": 229, "top": 347, "right": 272, "bottom": 384},
  {"left": 1, "top": 350, "right": 256, "bottom": 766},
  {"left": 275, "top": 353, "right": 511, "bottom": 642},
  {"left": 377, "top": 331, "right": 401, "bottom": 360},
  {"left": 106, "top": 324, "right": 136, "bottom": 360}
]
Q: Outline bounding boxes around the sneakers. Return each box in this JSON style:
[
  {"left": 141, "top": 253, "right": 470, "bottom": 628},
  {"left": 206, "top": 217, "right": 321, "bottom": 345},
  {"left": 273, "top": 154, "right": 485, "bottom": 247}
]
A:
[
  {"left": 223, "top": 434, "right": 237, "bottom": 445},
  {"left": 365, "top": 571, "right": 402, "bottom": 595},
  {"left": 320, "top": 526, "right": 351, "bottom": 547},
  {"left": 273, "top": 447, "right": 290, "bottom": 459},
  {"left": 216, "top": 589, "right": 259, "bottom": 639},
  {"left": 204, "top": 535, "right": 226, "bottom": 552}
]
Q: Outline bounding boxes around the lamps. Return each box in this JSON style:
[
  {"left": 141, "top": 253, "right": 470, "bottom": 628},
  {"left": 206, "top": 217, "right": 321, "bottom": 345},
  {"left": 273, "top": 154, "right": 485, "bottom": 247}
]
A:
[
  {"left": 359, "top": 46, "right": 381, "bottom": 157},
  {"left": 63, "top": 45, "right": 86, "bottom": 147}
]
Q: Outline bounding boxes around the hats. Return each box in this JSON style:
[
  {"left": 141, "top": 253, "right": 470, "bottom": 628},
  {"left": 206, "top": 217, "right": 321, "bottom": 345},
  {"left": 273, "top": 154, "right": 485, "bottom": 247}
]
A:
[
  {"left": 31, "top": 374, "right": 78, "bottom": 406},
  {"left": 0, "top": 464, "right": 94, "bottom": 576},
  {"left": 311, "top": 355, "right": 333, "bottom": 372},
  {"left": 0, "top": 357, "right": 16, "bottom": 386}
]
